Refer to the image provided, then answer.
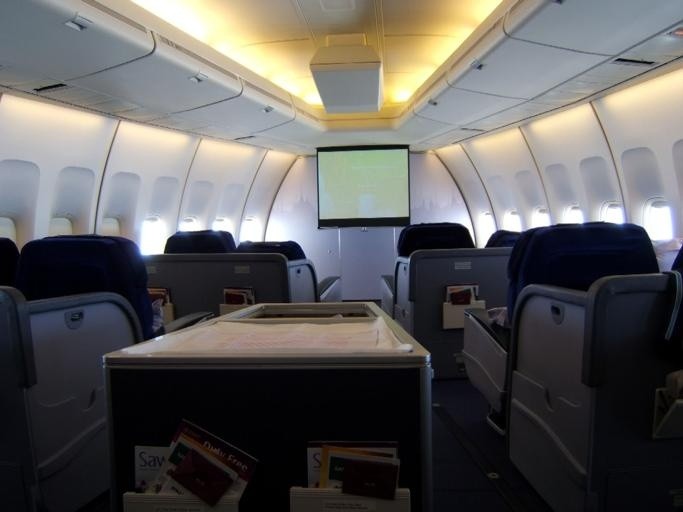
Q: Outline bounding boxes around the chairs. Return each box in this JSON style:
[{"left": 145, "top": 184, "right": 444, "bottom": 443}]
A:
[
  {"left": 0, "top": 230, "right": 342, "bottom": 512},
  {"left": 380, "top": 223, "right": 683, "bottom": 512}
]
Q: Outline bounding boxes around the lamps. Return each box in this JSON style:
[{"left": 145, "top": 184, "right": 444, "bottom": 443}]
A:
[{"left": 309, "top": 32, "right": 384, "bottom": 115}]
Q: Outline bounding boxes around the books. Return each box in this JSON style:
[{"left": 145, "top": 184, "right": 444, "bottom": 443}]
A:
[
  {"left": 157, "top": 449, "right": 235, "bottom": 509},
  {"left": 156, "top": 433, "right": 239, "bottom": 493},
  {"left": 655, "top": 398, "right": 682, "bottom": 439},
  {"left": 148, "top": 291, "right": 165, "bottom": 307},
  {"left": 223, "top": 287, "right": 255, "bottom": 305},
  {"left": 224, "top": 291, "right": 247, "bottom": 305},
  {"left": 325, "top": 455, "right": 398, "bottom": 503},
  {"left": 653, "top": 388, "right": 681, "bottom": 435},
  {"left": 148, "top": 420, "right": 262, "bottom": 499},
  {"left": 318, "top": 443, "right": 394, "bottom": 491},
  {"left": 304, "top": 438, "right": 400, "bottom": 491},
  {"left": 145, "top": 287, "right": 168, "bottom": 306},
  {"left": 444, "top": 284, "right": 479, "bottom": 304},
  {"left": 449, "top": 287, "right": 474, "bottom": 305}
]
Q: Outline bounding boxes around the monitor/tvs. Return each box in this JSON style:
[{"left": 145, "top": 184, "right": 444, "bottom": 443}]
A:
[{"left": 317, "top": 145, "right": 411, "bottom": 228}]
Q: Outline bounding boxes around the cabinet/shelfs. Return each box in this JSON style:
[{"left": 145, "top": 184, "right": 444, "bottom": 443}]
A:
[{"left": 101, "top": 301, "right": 434, "bottom": 512}]
